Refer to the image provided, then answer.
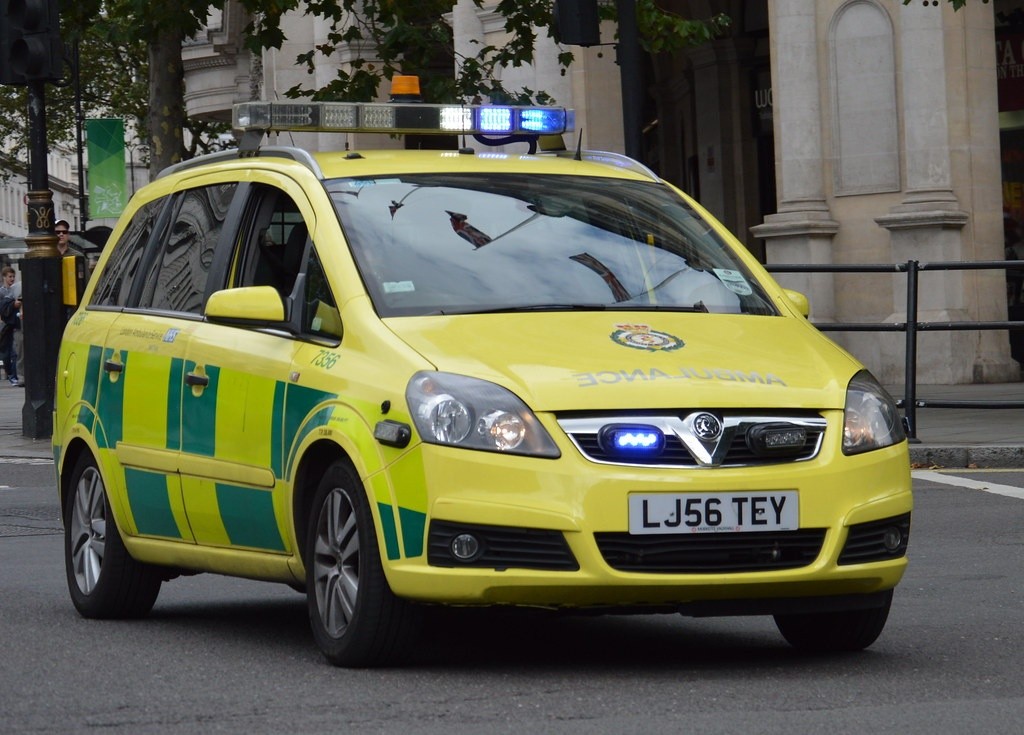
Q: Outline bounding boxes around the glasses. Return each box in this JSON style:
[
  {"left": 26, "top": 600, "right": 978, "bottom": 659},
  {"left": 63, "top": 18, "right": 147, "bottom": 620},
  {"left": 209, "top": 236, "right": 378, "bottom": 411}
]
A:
[{"left": 55, "top": 230, "right": 68, "bottom": 234}]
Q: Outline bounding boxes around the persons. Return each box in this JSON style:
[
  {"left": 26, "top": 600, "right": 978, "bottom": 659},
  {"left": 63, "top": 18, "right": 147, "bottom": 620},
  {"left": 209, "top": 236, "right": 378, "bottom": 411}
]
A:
[
  {"left": 54, "top": 219, "right": 90, "bottom": 287},
  {"left": 0, "top": 266, "right": 22, "bottom": 387}
]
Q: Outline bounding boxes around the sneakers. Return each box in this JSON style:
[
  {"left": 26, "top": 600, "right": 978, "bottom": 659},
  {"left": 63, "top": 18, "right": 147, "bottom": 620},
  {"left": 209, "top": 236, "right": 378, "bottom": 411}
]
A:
[{"left": 9, "top": 377, "right": 18, "bottom": 386}]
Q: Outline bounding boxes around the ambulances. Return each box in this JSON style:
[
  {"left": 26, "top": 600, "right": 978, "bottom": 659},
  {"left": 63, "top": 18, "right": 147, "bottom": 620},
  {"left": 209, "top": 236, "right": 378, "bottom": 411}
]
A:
[{"left": 48, "top": 73, "right": 915, "bottom": 673}]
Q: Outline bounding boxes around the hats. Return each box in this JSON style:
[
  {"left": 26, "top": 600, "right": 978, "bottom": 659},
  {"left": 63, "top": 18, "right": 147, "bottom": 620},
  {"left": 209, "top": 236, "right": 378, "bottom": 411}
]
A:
[{"left": 55, "top": 219, "right": 70, "bottom": 229}]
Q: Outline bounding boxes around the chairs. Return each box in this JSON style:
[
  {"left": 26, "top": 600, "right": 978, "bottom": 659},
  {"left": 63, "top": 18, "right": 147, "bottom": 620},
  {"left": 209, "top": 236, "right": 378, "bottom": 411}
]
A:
[{"left": 282, "top": 222, "right": 308, "bottom": 292}]
